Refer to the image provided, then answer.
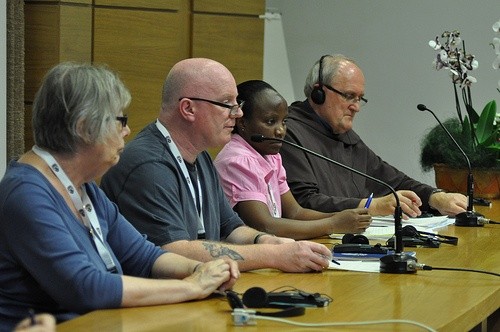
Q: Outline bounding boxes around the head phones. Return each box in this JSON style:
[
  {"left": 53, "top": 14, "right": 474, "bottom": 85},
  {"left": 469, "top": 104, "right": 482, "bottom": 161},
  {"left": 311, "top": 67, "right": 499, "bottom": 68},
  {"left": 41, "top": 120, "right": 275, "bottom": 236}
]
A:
[
  {"left": 226, "top": 287, "right": 330, "bottom": 317},
  {"left": 311, "top": 54, "right": 332, "bottom": 104},
  {"left": 333, "top": 232, "right": 387, "bottom": 254},
  {"left": 387, "top": 225, "right": 458, "bottom": 246}
]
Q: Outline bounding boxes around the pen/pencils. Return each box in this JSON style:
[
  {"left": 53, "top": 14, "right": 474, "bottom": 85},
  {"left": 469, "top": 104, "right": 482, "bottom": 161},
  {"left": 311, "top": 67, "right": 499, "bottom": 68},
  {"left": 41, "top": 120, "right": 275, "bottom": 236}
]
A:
[
  {"left": 411, "top": 199, "right": 416, "bottom": 202},
  {"left": 28, "top": 309, "right": 36, "bottom": 324},
  {"left": 319, "top": 254, "right": 341, "bottom": 265},
  {"left": 364, "top": 193, "right": 374, "bottom": 209}
]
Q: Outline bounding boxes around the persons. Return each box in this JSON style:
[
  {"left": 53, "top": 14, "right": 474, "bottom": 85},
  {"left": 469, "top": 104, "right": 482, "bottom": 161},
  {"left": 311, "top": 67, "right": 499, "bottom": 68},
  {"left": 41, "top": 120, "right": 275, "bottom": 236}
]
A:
[
  {"left": 280, "top": 54, "right": 475, "bottom": 220},
  {"left": 100, "top": 58, "right": 332, "bottom": 273},
  {"left": 0, "top": 61, "right": 240, "bottom": 332},
  {"left": 214, "top": 80, "right": 372, "bottom": 239}
]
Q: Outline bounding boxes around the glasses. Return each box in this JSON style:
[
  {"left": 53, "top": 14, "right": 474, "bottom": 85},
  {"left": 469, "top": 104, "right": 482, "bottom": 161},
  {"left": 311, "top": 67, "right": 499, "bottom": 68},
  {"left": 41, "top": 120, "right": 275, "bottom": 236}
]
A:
[
  {"left": 179, "top": 96, "right": 245, "bottom": 116},
  {"left": 116, "top": 114, "right": 127, "bottom": 127},
  {"left": 316, "top": 82, "right": 368, "bottom": 107}
]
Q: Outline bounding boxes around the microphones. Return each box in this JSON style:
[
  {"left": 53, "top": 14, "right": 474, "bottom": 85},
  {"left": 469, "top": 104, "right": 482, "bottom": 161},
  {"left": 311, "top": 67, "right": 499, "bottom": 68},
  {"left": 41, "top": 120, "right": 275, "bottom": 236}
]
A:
[
  {"left": 251, "top": 133, "right": 418, "bottom": 274},
  {"left": 417, "top": 103, "right": 488, "bottom": 227}
]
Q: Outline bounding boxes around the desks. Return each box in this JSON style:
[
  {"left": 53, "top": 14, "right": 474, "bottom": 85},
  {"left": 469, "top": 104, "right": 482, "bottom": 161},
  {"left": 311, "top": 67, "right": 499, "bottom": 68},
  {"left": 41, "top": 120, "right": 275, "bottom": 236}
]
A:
[{"left": 56, "top": 197, "right": 500, "bottom": 332}]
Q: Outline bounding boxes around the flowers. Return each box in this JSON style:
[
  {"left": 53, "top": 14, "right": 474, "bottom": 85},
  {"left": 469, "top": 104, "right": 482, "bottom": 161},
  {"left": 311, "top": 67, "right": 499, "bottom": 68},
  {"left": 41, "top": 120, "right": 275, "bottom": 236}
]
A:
[{"left": 429, "top": 20, "right": 500, "bottom": 158}]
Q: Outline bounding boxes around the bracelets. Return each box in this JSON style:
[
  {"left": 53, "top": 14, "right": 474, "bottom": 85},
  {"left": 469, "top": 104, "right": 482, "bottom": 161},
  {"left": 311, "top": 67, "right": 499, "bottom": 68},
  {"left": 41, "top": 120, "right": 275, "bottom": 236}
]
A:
[
  {"left": 193, "top": 264, "right": 200, "bottom": 273},
  {"left": 254, "top": 234, "right": 265, "bottom": 244}
]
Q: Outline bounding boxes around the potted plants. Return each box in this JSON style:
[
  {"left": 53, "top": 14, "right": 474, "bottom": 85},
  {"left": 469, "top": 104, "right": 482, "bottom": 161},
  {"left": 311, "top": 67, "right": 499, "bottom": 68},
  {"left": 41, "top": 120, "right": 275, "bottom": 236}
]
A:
[{"left": 420, "top": 101, "right": 500, "bottom": 199}]
